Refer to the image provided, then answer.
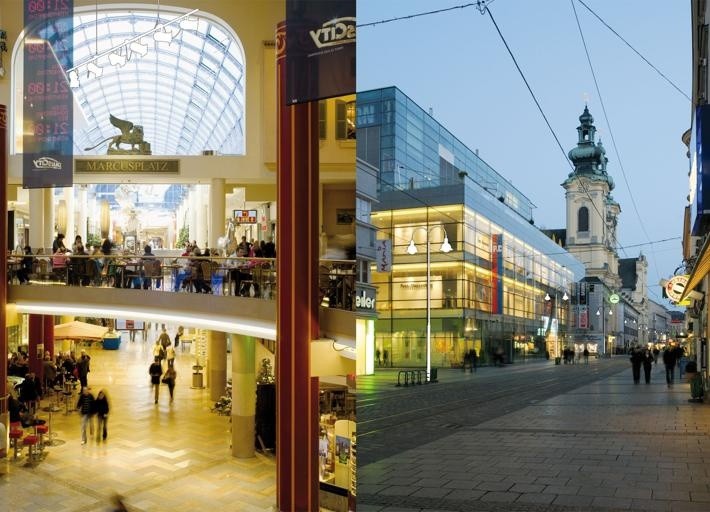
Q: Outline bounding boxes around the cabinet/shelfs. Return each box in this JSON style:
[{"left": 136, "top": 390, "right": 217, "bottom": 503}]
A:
[{"left": 319, "top": 412, "right": 356, "bottom": 499}]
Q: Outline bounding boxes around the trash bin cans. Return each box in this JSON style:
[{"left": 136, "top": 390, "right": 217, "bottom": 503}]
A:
[
  {"left": 192, "top": 366, "right": 203, "bottom": 387},
  {"left": 555, "top": 357, "right": 561, "bottom": 365},
  {"left": 686, "top": 361, "right": 697, "bottom": 382}
]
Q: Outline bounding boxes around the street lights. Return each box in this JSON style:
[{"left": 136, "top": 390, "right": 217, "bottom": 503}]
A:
[
  {"left": 404, "top": 226, "right": 454, "bottom": 381},
  {"left": 596, "top": 305, "right": 613, "bottom": 356},
  {"left": 638, "top": 324, "right": 669, "bottom": 350},
  {"left": 624, "top": 317, "right": 636, "bottom": 352},
  {"left": 544, "top": 285, "right": 569, "bottom": 363}
]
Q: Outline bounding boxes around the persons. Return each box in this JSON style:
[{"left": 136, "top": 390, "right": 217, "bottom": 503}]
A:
[
  {"left": 54, "top": 233, "right": 275, "bottom": 298},
  {"left": 16, "top": 246, "right": 35, "bottom": 285},
  {"left": 469, "top": 345, "right": 478, "bottom": 373},
  {"left": 382, "top": 349, "right": 388, "bottom": 368},
  {"left": 583, "top": 349, "right": 589, "bottom": 364},
  {"left": 95, "top": 391, "right": 109, "bottom": 443},
  {"left": 376, "top": 347, "right": 380, "bottom": 368},
  {"left": 149, "top": 325, "right": 184, "bottom": 405},
  {"left": 563, "top": 347, "right": 574, "bottom": 364},
  {"left": 632, "top": 342, "right": 683, "bottom": 386},
  {"left": 76, "top": 386, "right": 94, "bottom": 445},
  {"left": 8, "top": 347, "right": 90, "bottom": 422}
]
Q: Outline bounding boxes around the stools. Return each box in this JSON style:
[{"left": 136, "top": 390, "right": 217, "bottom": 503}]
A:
[{"left": 7, "top": 387, "right": 73, "bottom": 468}]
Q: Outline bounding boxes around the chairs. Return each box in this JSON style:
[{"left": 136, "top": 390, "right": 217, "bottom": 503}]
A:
[{"left": 6, "top": 251, "right": 355, "bottom": 311}]
